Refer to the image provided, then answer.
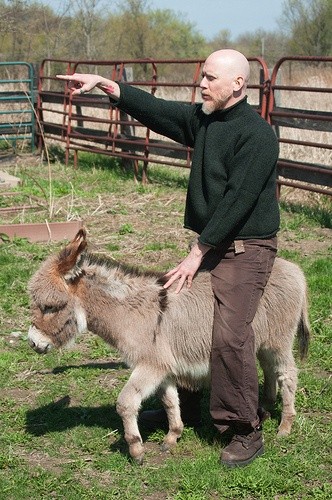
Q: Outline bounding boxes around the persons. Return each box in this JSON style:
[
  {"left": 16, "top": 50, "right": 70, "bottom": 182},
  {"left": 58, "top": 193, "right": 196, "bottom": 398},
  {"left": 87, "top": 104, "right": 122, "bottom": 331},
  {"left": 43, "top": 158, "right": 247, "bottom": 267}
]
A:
[{"left": 54, "top": 47, "right": 283, "bottom": 471}]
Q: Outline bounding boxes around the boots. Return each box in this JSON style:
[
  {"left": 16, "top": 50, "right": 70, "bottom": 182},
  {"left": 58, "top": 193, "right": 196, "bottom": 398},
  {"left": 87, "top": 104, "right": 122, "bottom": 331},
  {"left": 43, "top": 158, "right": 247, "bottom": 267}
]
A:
[{"left": 220, "top": 409, "right": 270, "bottom": 466}]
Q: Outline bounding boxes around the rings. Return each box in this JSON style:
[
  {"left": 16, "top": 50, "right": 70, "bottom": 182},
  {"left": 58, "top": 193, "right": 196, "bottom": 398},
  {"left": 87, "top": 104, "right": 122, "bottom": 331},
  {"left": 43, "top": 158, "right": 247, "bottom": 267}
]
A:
[{"left": 79, "top": 87, "right": 85, "bottom": 95}]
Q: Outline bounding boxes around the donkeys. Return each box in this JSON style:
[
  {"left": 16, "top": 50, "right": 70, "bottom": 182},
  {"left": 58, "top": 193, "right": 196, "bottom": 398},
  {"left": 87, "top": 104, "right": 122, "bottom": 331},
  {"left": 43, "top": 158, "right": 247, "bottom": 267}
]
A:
[{"left": 26, "top": 228, "right": 312, "bottom": 466}]
